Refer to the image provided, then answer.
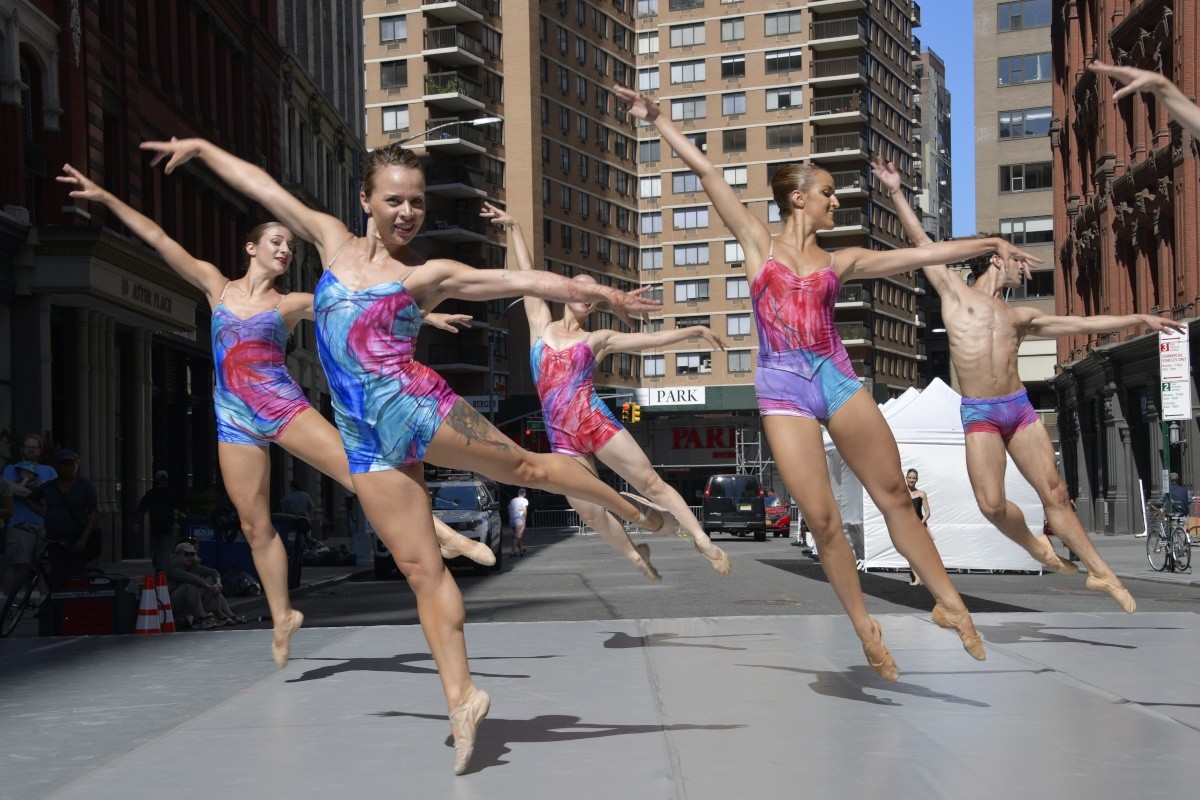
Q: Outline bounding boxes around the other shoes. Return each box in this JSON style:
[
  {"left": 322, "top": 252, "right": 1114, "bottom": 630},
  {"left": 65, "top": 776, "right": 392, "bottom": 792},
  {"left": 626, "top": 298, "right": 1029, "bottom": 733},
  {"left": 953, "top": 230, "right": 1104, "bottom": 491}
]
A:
[
  {"left": 510, "top": 554, "right": 519, "bottom": 557},
  {"left": 520, "top": 549, "right": 527, "bottom": 557},
  {"left": 192, "top": 615, "right": 227, "bottom": 628},
  {"left": 220, "top": 615, "right": 246, "bottom": 625}
]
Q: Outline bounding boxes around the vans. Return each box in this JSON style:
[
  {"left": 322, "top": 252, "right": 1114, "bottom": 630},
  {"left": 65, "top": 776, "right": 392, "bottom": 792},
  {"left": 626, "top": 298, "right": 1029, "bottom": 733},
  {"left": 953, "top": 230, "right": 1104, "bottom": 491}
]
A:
[{"left": 701, "top": 473, "right": 768, "bottom": 541}]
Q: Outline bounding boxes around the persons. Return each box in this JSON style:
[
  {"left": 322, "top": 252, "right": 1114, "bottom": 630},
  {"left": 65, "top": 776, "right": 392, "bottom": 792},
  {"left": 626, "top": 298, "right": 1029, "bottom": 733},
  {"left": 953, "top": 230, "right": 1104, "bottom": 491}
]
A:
[
  {"left": 612, "top": 85, "right": 1046, "bottom": 684},
  {"left": 1169, "top": 472, "right": 1189, "bottom": 520},
  {"left": 1088, "top": 57, "right": 1200, "bottom": 140},
  {"left": 766, "top": 487, "right": 784, "bottom": 508},
  {"left": 479, "top": 197, "right": 731, "bottom": 581},
  {"left": 900, "top": 469, "right": 930, "bottom": 587},
  {"left": 142, "top": 133, "right": 680, "bottom": 774},
  {"left": 0, "top": 430, "right": 357, "bottom": 629},
  {"left": 53, "top": 159, "right": 495, "bottom": 666},
  {"left": 869, "top": 154, "right": 1187, "bottom": 615},
  {"left": 507, "top": 488, "right": 530, "bottom": 559}
]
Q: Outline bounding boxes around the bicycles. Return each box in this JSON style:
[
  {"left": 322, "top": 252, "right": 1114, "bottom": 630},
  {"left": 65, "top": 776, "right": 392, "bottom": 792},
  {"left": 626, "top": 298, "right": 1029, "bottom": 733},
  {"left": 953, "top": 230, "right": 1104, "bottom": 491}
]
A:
[
  {"left": 1145, "top": 501, "right": 1192, "bottom": 572},
  {"left": 0, "top": 524, "right": 80, "bottom": 638}
]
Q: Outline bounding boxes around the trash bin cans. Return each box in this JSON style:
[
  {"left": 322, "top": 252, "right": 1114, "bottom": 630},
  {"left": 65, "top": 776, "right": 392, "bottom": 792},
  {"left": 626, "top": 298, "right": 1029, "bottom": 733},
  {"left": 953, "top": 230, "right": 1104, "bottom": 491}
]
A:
[{"left": 177, "top": 513, "right": 310, "bottom": 593}]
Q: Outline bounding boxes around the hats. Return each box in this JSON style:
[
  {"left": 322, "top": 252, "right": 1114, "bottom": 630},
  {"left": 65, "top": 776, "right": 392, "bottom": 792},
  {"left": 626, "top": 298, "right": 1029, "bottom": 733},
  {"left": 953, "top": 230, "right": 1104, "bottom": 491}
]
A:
[
  {"left": 14, "top": 463, "right": 39, "bottom": 476},
  {"left": 1170, "top": 472, "right": 1178, "bottom": 480},
  {"left": 176, "top": 542, "right": 197, "bottom": 553},
  {"left": 57, "top": 450, "right": 78, "bottom": 460},
  {"left": 155, "top": 470, "right": 168, "bottom": 480}
]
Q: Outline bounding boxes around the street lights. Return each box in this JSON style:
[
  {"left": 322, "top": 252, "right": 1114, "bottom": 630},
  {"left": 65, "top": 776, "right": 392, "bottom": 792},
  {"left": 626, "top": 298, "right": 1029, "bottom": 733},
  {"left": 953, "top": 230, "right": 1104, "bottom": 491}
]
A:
[{"left": 358, "top": 115, "right": 506, "bottom": 242}]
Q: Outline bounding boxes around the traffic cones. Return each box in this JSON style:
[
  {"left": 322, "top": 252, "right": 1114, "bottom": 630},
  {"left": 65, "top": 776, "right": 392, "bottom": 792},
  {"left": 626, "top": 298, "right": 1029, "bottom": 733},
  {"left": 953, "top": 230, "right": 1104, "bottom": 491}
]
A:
[{"left": 133, "top": 571, "right": 176, "bottom": 634}]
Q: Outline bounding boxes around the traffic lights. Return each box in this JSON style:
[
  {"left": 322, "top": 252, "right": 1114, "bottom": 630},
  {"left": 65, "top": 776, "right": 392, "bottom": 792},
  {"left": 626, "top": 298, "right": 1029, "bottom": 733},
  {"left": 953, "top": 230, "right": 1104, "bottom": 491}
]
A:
[
  {"left": 632, "top": 402, "right": 642, "bottom": 424},
  {"left": 621, "top": 403, "right": 630, "bottom": 422}
]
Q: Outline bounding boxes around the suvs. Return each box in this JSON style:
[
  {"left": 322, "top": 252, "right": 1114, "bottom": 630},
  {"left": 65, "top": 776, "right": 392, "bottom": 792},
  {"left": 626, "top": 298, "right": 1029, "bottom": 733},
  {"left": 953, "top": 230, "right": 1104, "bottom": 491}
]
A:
[{"left": 371, "top": 480, "right": 504, "bottom": 579}]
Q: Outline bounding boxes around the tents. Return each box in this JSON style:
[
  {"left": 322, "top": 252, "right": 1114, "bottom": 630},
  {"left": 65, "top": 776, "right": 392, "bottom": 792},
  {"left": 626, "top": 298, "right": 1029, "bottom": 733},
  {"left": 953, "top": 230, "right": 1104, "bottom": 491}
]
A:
[{"left": 796, "top": 376, "right": 1046, "bottom": 577}]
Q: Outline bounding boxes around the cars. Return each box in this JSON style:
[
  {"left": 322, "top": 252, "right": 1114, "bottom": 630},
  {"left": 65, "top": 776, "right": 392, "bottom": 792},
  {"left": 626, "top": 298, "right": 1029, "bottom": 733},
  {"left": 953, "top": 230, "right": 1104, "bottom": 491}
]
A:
[{"left": 762, "top": 492, "right": 792, "bottom": 537}]
